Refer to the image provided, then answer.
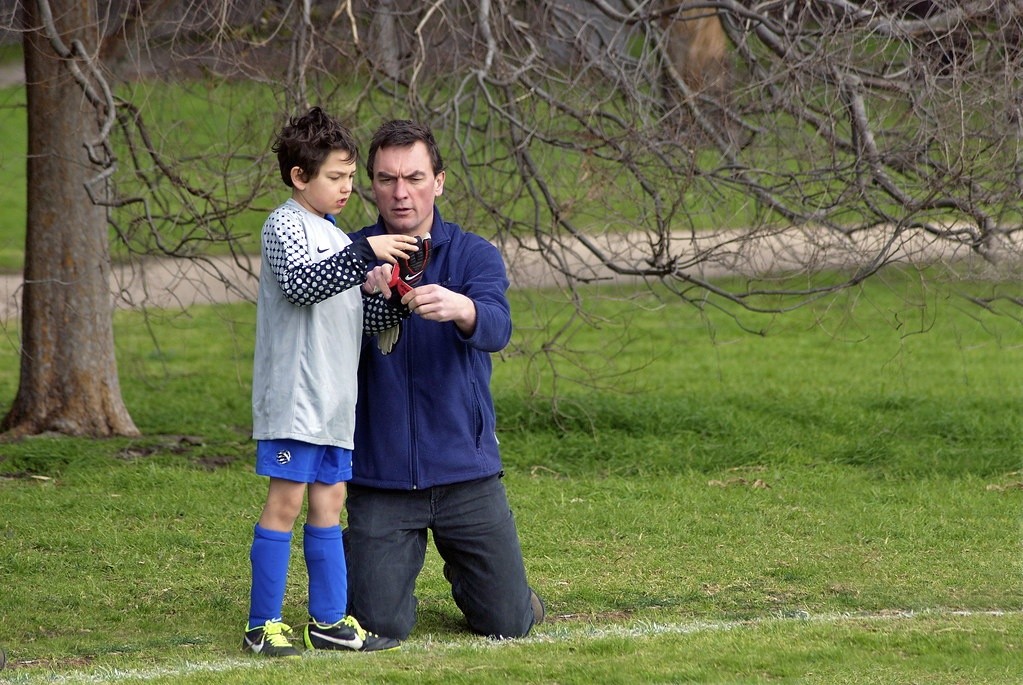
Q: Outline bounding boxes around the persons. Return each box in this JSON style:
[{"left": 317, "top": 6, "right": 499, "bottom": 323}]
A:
[
  {"left": 241, "top": 107, "right": 419, "bottom": 659},
  {"left": 346, "top": 119, "right": 545, "bottom": 641}
]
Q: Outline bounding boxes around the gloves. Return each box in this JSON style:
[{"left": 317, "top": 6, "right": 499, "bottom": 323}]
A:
[{"left": 388, "top": 234, "right": 432, "bottom": 297}]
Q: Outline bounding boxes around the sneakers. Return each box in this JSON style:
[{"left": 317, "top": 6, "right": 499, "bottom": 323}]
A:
[
  {"left": 241, "top": 620, "right": 302, "bottom": 660},
  {"left": 303, "top": 615, "right": 403, "bottom": 653},
  {"left": 529, "top": 588, "right": 545, "bottom": 625}
]
